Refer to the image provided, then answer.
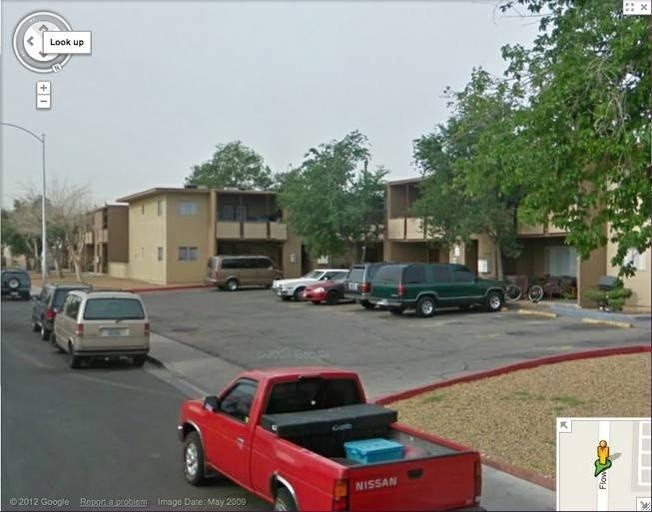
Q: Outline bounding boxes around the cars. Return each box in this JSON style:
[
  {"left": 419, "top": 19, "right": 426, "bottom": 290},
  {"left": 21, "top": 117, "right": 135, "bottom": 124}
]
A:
[
  {"left": 272, "top": 268, "right": 349, "bottom": 302},
  {"left": 301, "top": 272, "right": 349, "bottom": 305}
]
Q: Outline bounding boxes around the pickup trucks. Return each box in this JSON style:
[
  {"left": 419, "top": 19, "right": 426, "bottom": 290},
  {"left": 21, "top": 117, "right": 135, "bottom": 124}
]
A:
[{"left": 176, "top": 368, "right": 484, "bottom": 511}]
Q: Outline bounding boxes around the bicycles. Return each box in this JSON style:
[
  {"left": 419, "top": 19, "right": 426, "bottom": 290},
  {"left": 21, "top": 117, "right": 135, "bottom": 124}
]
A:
[{"left": 505, "top": 279, "right": 544, "bottom": 304}]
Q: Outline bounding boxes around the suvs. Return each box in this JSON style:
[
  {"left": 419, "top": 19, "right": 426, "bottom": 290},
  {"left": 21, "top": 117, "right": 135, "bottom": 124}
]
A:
[{"left": 1, "top": 267, "right": 32, "bottom": 301}]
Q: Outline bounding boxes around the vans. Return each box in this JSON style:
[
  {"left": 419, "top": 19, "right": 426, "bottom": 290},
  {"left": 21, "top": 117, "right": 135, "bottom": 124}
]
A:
[
  {"left": 49, "top": 288, "right": 151, "bottom": 369},
  {"left": 343, "top": 260, "right": 399, "bottom": 310},
  {"left": 31, "top": 279, "right": 94, "bottom": 342},
  {"left": 203, "top": 254, "right": 284, "bottom": 291},
  {"left": 369, "top": 261, "right": 511, "bottom": 318}
]
